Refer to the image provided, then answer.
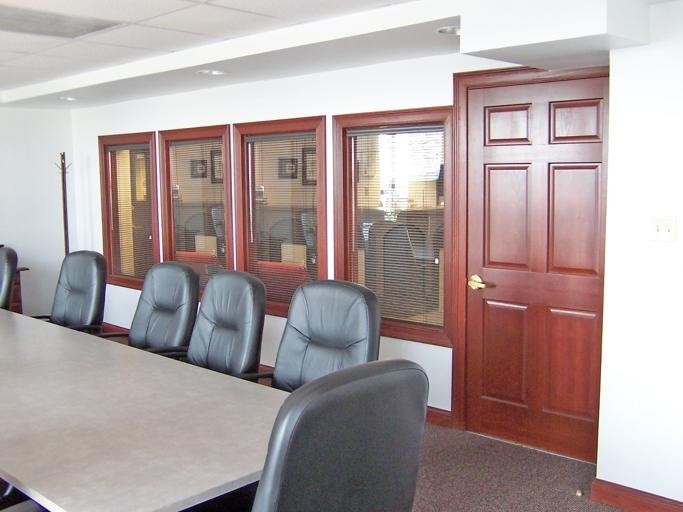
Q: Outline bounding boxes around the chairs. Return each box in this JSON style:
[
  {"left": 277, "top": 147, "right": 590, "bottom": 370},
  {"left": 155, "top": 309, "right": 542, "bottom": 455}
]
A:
[
  {"left": 150, "top": 272, "right": 266, "bottom": 385},
  {"left": 96, "top": 262, "right": 200, "bottom": 353},
  {"left": 0, "top": 246, "right": 17, "bottom": 309},
  {"left": 252, "top": 360, "right": 430, "bottom": 511},
  {"left": 255, "top": 260, "right": 308, "bottom": 306},
  {"left": 174, "top": 250, "right": 221, "bottom": 297},
  {"left": 30, "top": 250, "right": 106, "bottom": 335},
  {"left": 231, "top": 280, "right": 380, "bottom": 403}
]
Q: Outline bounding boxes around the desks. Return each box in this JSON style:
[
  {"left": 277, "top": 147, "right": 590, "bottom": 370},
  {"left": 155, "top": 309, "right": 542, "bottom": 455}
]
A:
[{"left": 1, "top": 309, "right": 291, "bottom": 510}]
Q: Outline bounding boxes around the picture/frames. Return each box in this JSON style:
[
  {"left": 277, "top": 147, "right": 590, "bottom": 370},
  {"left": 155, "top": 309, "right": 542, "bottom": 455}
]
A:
[
  {"left": 277, "top": 146, "right": 316, "bottom": 185},
  {"left": 190, "top": 150, "right": 222, "bottom": 183}
]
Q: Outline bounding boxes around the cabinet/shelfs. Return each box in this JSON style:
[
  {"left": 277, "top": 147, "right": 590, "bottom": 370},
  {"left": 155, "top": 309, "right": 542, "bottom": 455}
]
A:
[{"left": 14, "top": 271, "right": 22, "bottom": 314}]
